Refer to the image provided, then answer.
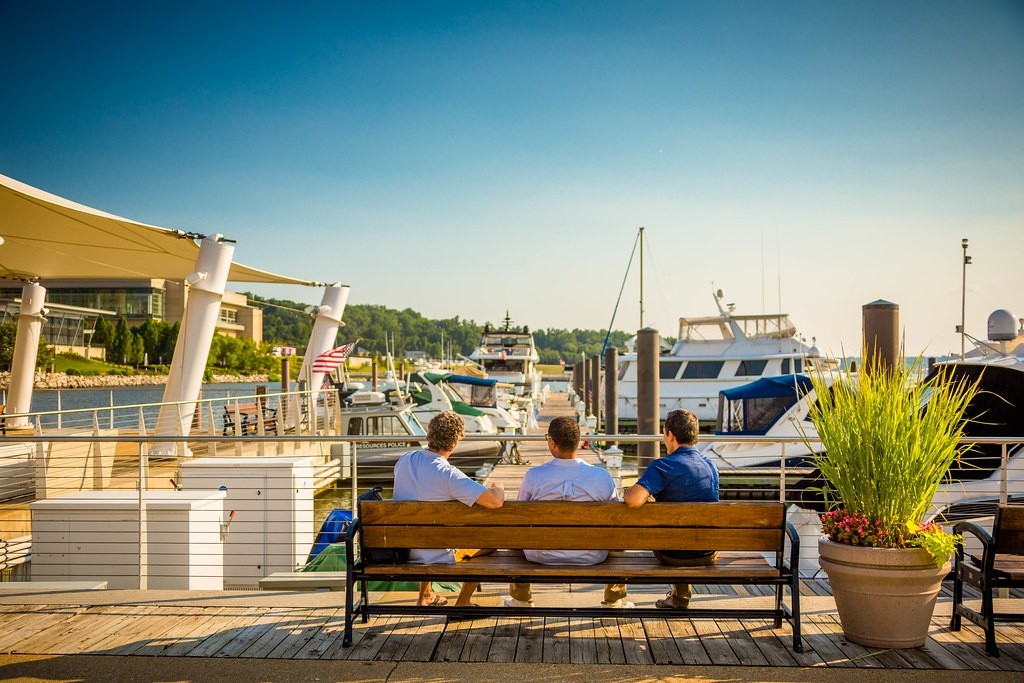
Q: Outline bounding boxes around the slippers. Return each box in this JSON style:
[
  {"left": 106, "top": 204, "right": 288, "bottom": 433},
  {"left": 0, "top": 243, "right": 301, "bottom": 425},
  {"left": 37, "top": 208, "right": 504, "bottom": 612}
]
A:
[
  {"left": 473, "top": 603, "right": 479, "bottom": 606},
  {"left": 429, "top": 595, "right": 448, "bottom": 605}
]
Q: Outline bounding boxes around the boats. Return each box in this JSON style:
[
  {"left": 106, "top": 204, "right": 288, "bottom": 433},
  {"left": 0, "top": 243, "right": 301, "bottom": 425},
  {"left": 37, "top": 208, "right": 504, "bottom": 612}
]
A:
[
  {"left": 595, "top": 289, "right": 840, "bottom": 428},
  {"left": 326, "top": 365, "right": 502, "bottom": 482},
  {"left": 694, "top": 371, "right": 929, "bottom": 497},
  {"left": 780, "top": 239, "right": 1024, "bottom": 575},
  {"left": 447, "top": 374, "right": 524, "bottom": 430},
  {"left": 402, "top": 371, "right": 496, "bottom": 436},
  {"left": 467, "top": 310, "right": 544, "bottom": 396}
]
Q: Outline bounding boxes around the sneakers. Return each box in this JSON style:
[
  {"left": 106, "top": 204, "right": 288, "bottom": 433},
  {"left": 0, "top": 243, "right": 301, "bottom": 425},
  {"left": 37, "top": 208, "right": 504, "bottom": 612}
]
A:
[
  {"left": 655, "top": 599, "right": 688, "bottom": 608},
  {"left": 504, "top": 597, "right": 535, "bottom": 607},
  {"left": 601, "top": 599, "right": 635, "bottom": 608}
]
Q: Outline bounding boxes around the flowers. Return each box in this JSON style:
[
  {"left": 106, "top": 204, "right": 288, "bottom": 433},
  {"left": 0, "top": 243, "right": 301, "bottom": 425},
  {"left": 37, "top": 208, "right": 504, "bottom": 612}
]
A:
[{"left": 789, "top": 341, "right": 1014, "bottom": 567}]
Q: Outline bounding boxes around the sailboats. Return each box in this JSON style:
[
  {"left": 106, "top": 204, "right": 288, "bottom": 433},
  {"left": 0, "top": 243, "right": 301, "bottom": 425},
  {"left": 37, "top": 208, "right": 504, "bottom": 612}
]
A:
[{"left": 577, "top": 226, "right": 671, "bottom": 401}]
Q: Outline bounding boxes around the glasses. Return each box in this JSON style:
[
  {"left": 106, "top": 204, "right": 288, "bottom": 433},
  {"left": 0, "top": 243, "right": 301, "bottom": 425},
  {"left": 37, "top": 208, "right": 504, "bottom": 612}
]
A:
[{"left": 544, "top": 433, "right": 551, "bottom": 440}]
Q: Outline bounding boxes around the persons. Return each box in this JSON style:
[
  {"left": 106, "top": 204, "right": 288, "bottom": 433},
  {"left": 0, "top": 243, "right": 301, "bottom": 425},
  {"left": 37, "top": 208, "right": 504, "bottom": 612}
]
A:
[
  {"left": 624, "top": 409, "right": 719, "bottom": 609},
  {"left": 504, "top": 416, "right": 636, "bottom": 608},
  {"left": 394, "top": 410, "right": 505, "bottom": 621}
]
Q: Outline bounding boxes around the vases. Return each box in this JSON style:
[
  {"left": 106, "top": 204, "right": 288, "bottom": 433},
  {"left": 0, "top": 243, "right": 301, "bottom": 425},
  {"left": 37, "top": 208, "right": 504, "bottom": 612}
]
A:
[{"left": 817, "top": 535, "right": 953, "bottom": 648}]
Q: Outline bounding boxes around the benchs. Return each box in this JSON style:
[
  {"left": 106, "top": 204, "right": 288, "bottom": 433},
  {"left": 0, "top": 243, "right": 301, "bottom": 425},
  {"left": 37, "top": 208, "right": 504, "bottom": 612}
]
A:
[
  {"left": 949, "top": 504, "right": 1024, "bottom": 657},
  {"left": 222, "top": 402, "right": 278, "bottom": 435},
  {"left": 342, "top": 496, "right": 802, "bottom": 650}
]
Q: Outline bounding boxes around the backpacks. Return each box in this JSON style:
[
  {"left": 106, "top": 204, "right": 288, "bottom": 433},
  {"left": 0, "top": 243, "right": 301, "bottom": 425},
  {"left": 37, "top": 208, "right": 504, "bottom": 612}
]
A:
[{"left": 357, "top": 486, "right": 411, "bottom": 565}]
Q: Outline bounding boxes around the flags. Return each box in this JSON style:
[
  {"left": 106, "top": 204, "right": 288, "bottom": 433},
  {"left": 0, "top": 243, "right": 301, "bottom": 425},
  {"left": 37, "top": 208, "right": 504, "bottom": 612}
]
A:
[{"left": 312, "top": 341, "right": 355, "bottom": 374}]
